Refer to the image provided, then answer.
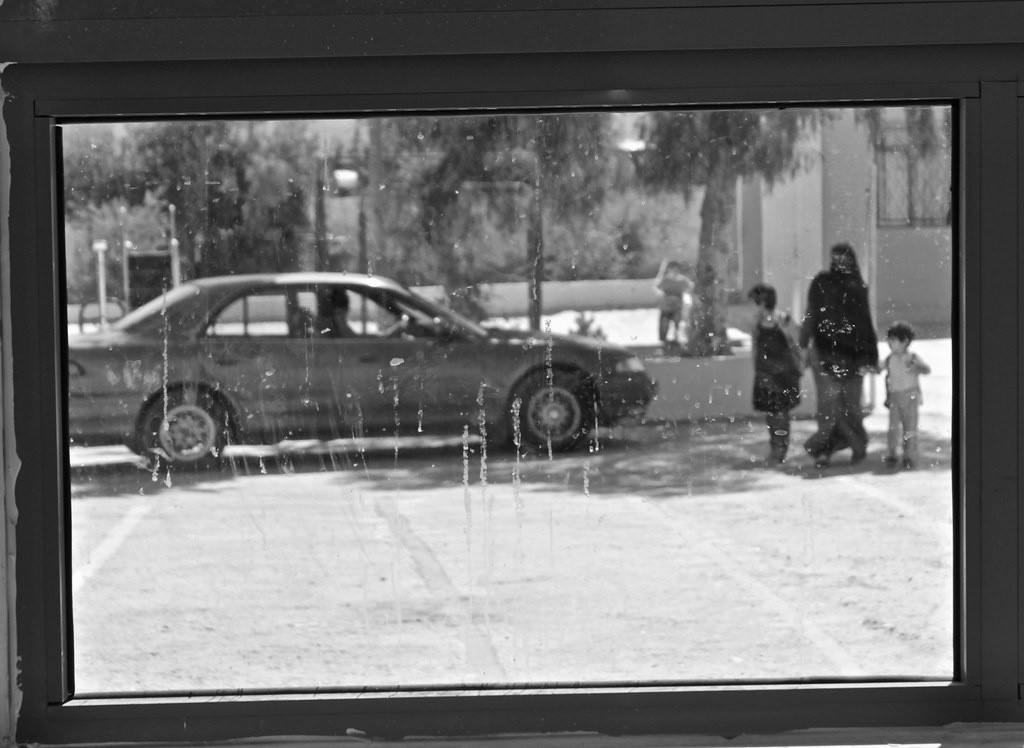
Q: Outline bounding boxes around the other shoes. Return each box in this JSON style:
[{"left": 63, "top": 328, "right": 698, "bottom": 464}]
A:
[
  {"left": 886, "top": 451, "right": 897, "bottom": 466},
  {"left": 815, "top": 454, "right": 830, "bottom": 470},
  {"left": 849, "top": 434, "right": 870, "bottom": 464},
  {"left": 903, "top": 457, "right": 915, "bottom": 470}
]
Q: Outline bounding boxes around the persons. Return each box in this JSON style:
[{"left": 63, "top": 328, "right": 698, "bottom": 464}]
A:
[
  {"left": 874, "top": 322, "right": 932, "bottom": 469},
  {"left": 656, "top": 259, "right": 693, "bottom": 342},
  {"left": 800, "top": 243, "right": 881, "bottom": 465},
  {"left": 748, "top": 285, "right": 811, "bottom": 463},
  {"left": 325, "top": 289, "right": 406, "bottom": 338}
]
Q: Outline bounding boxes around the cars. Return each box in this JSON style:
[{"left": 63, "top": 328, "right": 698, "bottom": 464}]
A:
[{"left": 65, "top": 272, "right": 662, "bottom": 478}]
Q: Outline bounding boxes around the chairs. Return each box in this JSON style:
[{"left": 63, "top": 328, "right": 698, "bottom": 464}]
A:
[{"left": 290, "top": 308, "right": 315, "bottom": 338}]
